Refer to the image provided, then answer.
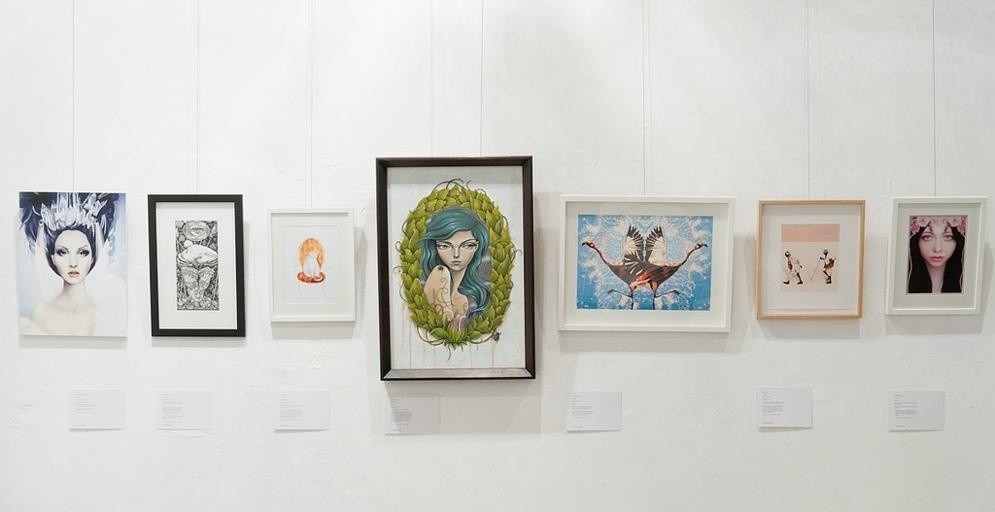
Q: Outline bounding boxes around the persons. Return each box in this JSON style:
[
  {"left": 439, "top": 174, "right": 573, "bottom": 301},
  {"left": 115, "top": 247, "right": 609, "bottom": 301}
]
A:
[
  {"left": 19, "top": 193, "right": 120, "bottom": 338},
  {"left": 419, "top": 206, "right": 492, "bottom": 328},
  {"left": 820, "top": 249, "right": 835, "bottom": 284},
  {"left": 783, "top": 251, "right": 804, "bottom": 284},
  {"left": 907, "top": 215, "right": 966, "bottom": 293}
]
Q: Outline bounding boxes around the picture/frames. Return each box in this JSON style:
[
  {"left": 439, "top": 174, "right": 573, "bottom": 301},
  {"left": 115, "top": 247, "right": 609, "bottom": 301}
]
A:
[
  {"left": 264, "top": 205, "right": 358, "bottom": 325},
  {"left": 145, "top": 193, "right": 246, "bottom": 337},
  {"left": 884, "top": 196, "right": 988, "bottom": 316},
  {"left": 372, "top": 154, "right": 538, "bottom": 381},
  {"left": 556, "top": 192, "right": 735, "bottom": 337},
  {"left": 755, "top": 198, "right": 866, "bottom": 321}
]
